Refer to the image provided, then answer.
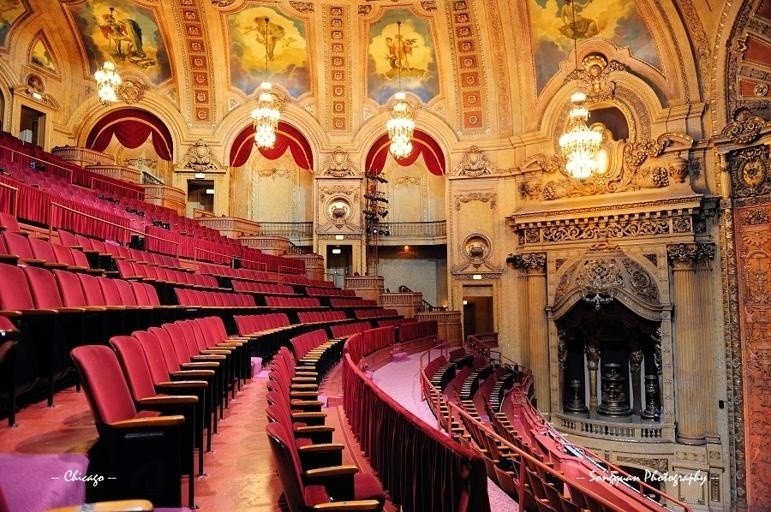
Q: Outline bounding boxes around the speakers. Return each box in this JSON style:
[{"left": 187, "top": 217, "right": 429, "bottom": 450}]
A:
[{"left": 719, "top": 400, "right": 724, "bottom": 409}]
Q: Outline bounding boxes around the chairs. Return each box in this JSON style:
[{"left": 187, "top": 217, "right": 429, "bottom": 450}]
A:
[{"left": 1, "top": 131, "right": 609, "bottom": 511}]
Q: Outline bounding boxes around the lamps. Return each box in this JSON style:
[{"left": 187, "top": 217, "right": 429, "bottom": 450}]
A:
[
  {"left": 249, "top": 18, "right": 282, "bottom": 150},
  {"left": 385, "top": 22, "right": 416, "bottom": 160},
  {"left": 93, "top": 6, "right": 123, "bottom": 103},
  {"left": 558, "top": 1, "right": 608, "bottom": 181}
]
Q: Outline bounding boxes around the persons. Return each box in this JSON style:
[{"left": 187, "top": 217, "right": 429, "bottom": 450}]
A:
[
  {"left": 101, "top": 0, "right": 602, "bottom": 72},
  {"left": 745, "top": 305, "right": 756, "bottom": 336}
]
